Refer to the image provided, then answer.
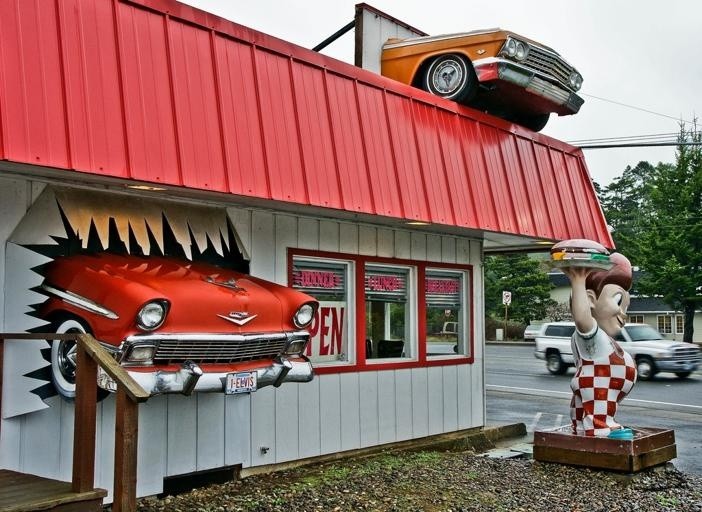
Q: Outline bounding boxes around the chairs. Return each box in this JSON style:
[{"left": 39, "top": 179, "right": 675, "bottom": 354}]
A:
[{"left": 366, "top": 339, "right": 405, "bottom": 359}]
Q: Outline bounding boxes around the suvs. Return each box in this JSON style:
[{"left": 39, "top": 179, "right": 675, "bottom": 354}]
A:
[{"left": 534, "top": 321, "right": 702, "bottom": 381}]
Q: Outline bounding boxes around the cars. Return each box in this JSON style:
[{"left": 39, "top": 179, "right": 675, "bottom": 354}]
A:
[
  {"left": 38, "top": 252, "right": 319, "bottom": 403},
  {"left": 523, "top": 325, "right": 539, "bottom": 341},
  {"left": 381, "top": 27, "right": 585, "bottom": 133}
]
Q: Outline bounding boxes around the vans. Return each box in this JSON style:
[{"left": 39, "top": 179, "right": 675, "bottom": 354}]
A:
[{"left": 442, "top": 322, "right": 458, "bottom": 337}]
